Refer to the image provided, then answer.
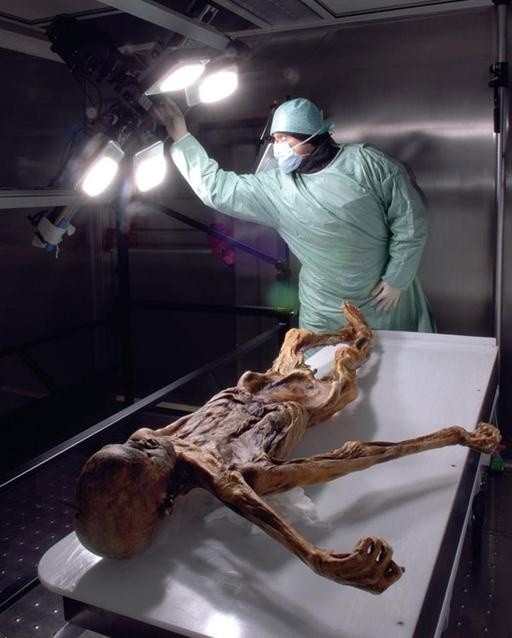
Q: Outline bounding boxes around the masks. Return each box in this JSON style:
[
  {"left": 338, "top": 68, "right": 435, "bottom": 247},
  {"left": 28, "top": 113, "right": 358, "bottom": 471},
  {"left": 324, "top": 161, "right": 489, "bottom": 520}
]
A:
[{"left": 273, "top": 142, "right": 303, "bottom": 174}]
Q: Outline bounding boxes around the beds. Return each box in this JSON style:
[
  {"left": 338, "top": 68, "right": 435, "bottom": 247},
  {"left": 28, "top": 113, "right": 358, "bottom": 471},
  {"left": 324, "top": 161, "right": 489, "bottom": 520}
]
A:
[{"left": 38, "top": 330, "right": 501, "bottom": 638}]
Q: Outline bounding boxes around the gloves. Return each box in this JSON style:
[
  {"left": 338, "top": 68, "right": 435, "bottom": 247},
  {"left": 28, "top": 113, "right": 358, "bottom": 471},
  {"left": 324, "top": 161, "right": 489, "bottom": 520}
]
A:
[
  {"left": 369, "top": 281, "right": 401, "bottom": 312},
  {"left": 152, "top": 95, "right": 188, "bottom": 141}
]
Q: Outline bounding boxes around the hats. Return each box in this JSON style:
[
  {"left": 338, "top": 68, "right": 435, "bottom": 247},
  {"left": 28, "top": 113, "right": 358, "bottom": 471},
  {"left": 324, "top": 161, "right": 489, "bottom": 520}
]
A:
[{"left": 270, "top": 97, "right": 328, "bottom": 136}]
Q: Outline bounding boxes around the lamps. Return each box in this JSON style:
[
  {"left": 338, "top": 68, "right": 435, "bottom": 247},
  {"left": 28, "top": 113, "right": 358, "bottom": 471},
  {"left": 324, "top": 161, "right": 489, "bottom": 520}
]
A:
[{"left": 72, "top": 48, "right": 240, "bottom": 198}]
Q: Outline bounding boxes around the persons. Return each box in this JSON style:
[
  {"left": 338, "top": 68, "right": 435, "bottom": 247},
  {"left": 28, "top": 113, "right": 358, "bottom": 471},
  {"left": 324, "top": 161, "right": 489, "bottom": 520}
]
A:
[{"left": 150, "top": 95, "right": 438, "bottom": 338}]
